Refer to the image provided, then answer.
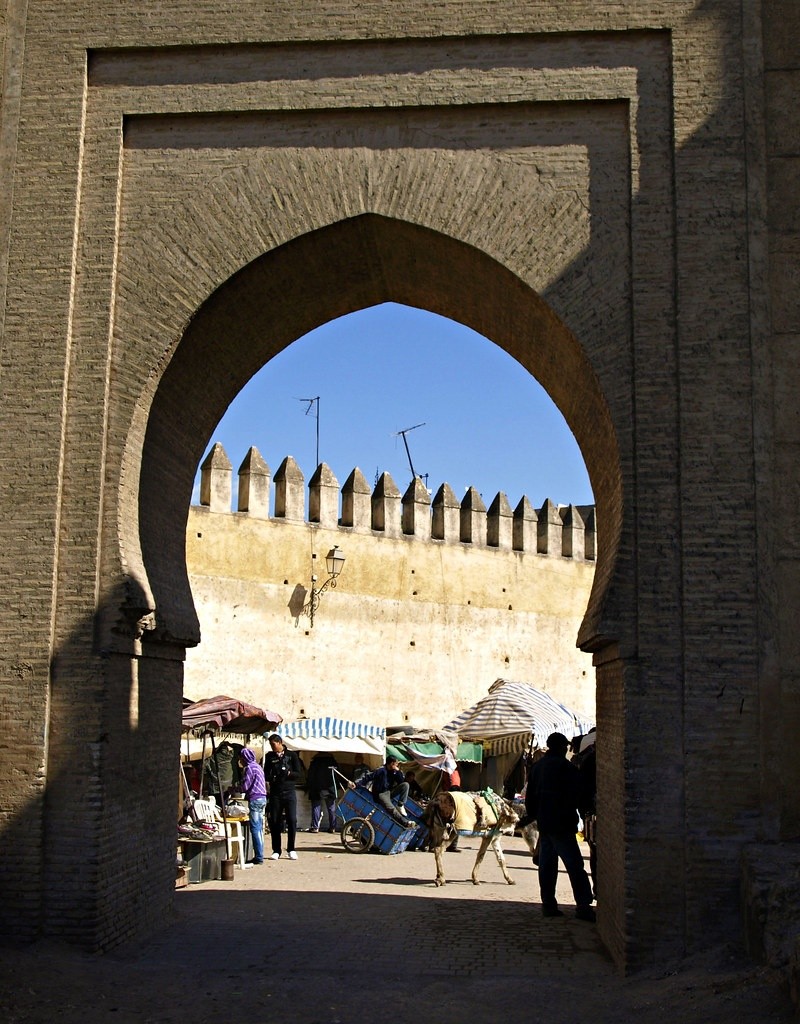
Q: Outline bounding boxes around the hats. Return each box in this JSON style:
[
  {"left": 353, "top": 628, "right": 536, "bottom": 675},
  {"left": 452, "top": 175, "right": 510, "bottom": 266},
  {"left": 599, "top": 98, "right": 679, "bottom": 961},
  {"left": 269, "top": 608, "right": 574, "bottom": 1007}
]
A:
[{"left": 547, "top": 733, "right": 572, "bottom": 747}]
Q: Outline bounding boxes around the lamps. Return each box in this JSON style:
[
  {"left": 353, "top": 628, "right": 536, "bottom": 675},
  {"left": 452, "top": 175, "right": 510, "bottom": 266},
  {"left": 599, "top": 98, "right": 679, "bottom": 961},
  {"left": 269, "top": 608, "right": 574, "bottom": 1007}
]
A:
[{"left": 310, "top": 544, "right": 347, "bottom": 628}]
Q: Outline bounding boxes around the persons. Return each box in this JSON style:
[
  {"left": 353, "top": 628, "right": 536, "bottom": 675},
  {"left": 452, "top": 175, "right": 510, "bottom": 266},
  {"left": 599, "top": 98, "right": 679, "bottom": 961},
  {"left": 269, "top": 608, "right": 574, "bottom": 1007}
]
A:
[
  {"left": 204, "top": 740, "right": 245, "bottom": 806},
  {"left": 350, "top": 756, "right": 416, "bottom": 828},
  {"left": 525, "top": 734, "right": 596, "bottom": 921},
  {"left": 404, "top": 770, "right": 426, "bottom": 801},
  {"left": 259, "top": 734, "right": 301, "bottom": 861},
  {"left": 231, "top": 748, "right": 267, "bottom": 864},
  {"left": 441, "top": 770, "right": 462, "bottom": 853},
  {"left": 352, "top": 753, "right": 371, "bottom": 782},
  {"left": 306, "top": 751, "right": 340, "bottom": 834}
]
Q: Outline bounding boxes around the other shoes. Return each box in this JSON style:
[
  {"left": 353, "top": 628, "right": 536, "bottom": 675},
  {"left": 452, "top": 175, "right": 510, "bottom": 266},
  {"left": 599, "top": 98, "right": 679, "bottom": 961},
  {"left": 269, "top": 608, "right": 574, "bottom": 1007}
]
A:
[
  {"left": 574, "top": 904, "right": 597, "bottom": 918},
  {"left": 419, "top": 844, "right": 426, "bottom": 852},
  {"left": 543, "top": 907, "right": 564, "bottom": 917},
  {"left": 250, "top": 857, "right": 263, "bottom": 862},
  {"left": 309, "top": 828, "right": 318, "bottom": 833},
  {"left": 407, "top": 821, "right": 415, "bottom": 827},
  {"left": 428, "top": 846, "right": 435, "bottom": 852},
  {"left": 327, "top": 828, "right": 335, "bottom": 833},
  {"left": 446, "top": 846, "right": 461, "bottom": 852},
  {"left": 396, "top": 804, "right": 407, "bottom": 816}
]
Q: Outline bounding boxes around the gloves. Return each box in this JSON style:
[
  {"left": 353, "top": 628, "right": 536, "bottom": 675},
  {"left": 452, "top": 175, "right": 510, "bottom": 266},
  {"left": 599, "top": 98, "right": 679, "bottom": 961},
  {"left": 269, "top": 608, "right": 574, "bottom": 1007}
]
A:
[{"left": 280, "top": 767, "right": 288, "bottom": 775}]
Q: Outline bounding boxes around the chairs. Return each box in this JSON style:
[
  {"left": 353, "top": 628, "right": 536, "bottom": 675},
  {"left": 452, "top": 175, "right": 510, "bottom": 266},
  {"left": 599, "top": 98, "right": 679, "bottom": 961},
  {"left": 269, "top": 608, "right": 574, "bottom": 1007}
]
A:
[{"left": 194, "top": 800, "right": 245, "bottom": 869}]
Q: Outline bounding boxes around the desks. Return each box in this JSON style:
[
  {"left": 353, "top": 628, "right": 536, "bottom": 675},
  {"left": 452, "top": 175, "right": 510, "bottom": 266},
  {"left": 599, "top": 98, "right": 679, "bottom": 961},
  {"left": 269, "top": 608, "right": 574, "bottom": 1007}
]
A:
[
  {"left": 210, "top": 817, "right": 253, "bottom": 863},
  {"left": 178, "top": 835, "right": 227, "bottom": 884}
]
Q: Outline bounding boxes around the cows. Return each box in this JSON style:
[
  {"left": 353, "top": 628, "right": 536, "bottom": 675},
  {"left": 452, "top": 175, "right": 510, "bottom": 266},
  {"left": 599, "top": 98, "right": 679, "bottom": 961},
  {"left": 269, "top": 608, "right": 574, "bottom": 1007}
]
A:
[{"left": 423, "top": 791, "right": 539, "bottom": 887}]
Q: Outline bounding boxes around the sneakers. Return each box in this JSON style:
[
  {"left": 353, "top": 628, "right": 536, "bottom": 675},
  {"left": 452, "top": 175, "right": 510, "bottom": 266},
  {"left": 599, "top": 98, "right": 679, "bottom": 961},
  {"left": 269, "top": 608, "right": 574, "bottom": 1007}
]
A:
[
  {"left": 271, "top": 852, "right": 280, "bottom": 859},
  {"left": 288, "top": 851, "right": 298, "bottom": 859}
]
